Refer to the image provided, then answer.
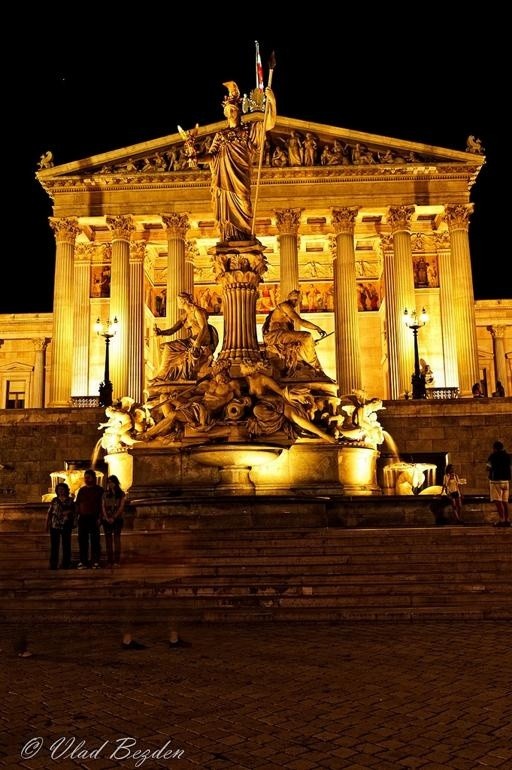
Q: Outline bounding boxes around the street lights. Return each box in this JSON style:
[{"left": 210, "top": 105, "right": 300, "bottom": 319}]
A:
[
  {"left": 401, "top": 305, "right": 429, "bottom": 399},
  {"left": 92, "top": 317, "right": 119, "bottom": 407}
]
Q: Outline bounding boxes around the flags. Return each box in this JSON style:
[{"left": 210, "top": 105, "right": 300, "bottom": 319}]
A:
[{"left": 255, "top": 40, "right": 265, "bottom": 93}]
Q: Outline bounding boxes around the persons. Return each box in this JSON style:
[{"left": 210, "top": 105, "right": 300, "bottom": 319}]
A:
[
  {"left": 89, "top": 254, "right": 438, "bottom": 444},
  {"left": 45, "top": 483, "right": 75, "bottom": 569},
  {"left": 492, "top": 381, "right": 505, "bottom": 397},
  {"left": 440, "top": 464, "right": 464, "bottom": 524},
  {"left": 486, "top": 441, "right": 512, "bottom": 527},
  {"left": 181, "top": 81, "right": 278, "bottom": 247},
  {"left": 472, "top": 383, "right": 485, "bottom": 398},
  {"left": 395, "top": 467, "right": 426, "bottom": 495},
  {"left": 74, "top": 470, "right": 106, "bottom": 570},
  {"left": 102, "top": 475, "right": 125, "bottom": 568}
]
